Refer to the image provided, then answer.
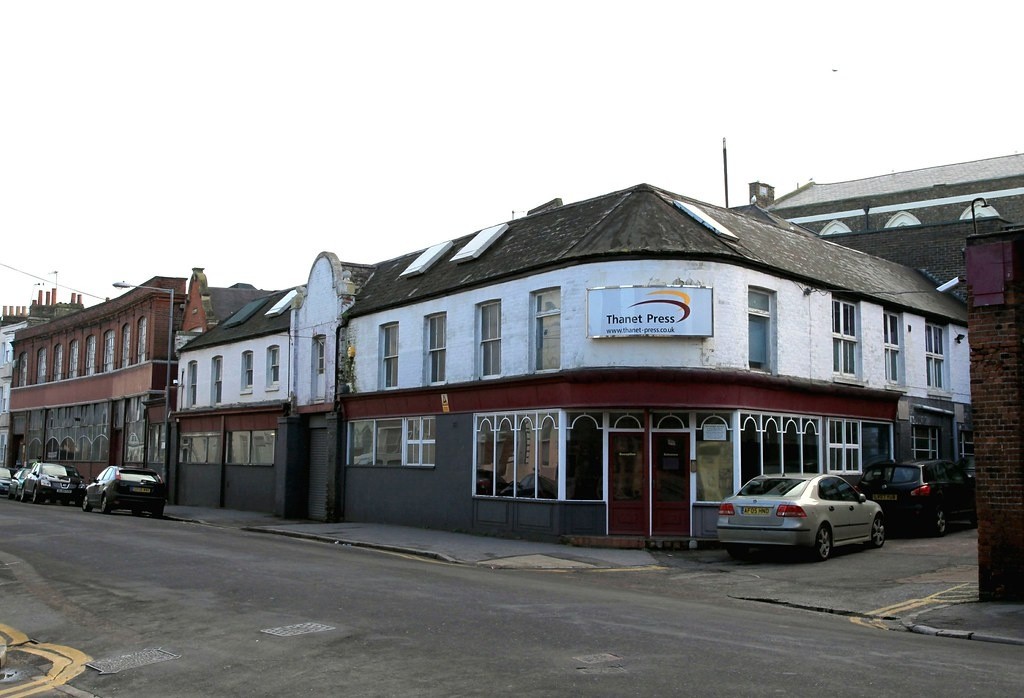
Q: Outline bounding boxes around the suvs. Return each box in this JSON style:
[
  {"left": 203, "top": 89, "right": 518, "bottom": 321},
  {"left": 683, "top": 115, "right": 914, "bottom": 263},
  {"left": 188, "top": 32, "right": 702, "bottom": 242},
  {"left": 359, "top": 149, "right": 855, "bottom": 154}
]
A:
[
  {"left": 854, "top": 459, "right": 977, "bottom": 536},
  {"left": 476, "top": 469, "right": 507, "bottom": 496}
]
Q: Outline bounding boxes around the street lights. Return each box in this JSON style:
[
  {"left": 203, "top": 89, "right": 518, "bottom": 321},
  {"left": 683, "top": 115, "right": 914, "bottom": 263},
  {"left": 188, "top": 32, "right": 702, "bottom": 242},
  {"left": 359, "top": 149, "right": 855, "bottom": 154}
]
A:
[{"left": 112, "top": 281, "right": 174, "bottom": 483}]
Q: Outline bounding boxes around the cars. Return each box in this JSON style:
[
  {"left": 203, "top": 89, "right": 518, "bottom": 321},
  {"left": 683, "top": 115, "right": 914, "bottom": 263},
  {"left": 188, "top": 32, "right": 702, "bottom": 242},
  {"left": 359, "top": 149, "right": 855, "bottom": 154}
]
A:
[
  {"left": 715, "top": 473, "right": 887, "bottom": 561},
  {"left": 501, "top": 474, "right": 557, "bottom": 499},
  {"left": 8, "top": 467, "right": 32, "bottom": 500},
  {"left": 0, "top": 467, "right": 17, "bottom": 494},
  {"left": 80, "top": 465, "right": 166, "bottom": 518},
  {"left": 21, "top": 462, "right": 86, "bottom": 507}
]
